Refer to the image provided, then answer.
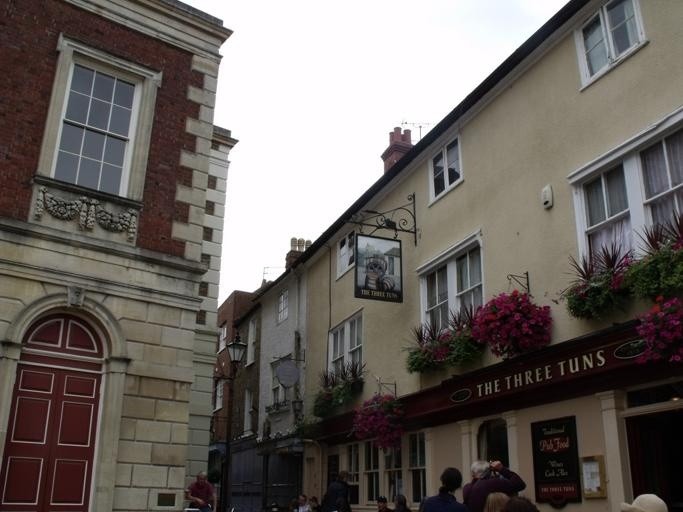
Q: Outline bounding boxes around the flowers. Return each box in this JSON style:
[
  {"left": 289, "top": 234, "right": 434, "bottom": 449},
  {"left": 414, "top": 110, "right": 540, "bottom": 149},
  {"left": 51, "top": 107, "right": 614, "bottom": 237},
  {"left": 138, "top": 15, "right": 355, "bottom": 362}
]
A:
[
  {"left": 563, "top": 208, "right": 683, "bottom": 365},
  {"left": 354, "top": 396, "right": 406, "bottom": 454},
  {"left": 404, "top": 287, "right": 555, "bottom": 375}
]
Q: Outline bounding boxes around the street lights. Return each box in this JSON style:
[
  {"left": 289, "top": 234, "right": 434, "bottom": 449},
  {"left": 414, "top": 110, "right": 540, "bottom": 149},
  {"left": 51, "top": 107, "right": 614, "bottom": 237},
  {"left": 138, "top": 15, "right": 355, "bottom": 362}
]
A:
[{"left": 218, "top": 332, "right": 247, "bottom": 511}]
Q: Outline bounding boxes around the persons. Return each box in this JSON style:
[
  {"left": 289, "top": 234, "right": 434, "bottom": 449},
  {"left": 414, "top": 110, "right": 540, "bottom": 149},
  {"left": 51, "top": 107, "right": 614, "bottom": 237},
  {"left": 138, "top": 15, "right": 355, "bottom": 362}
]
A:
[
  {"left": 184, "top": 470, "right": 217, "bottom": 512},
  {"left": 270, "top": 458, "right": 539, "bottom": 512}
]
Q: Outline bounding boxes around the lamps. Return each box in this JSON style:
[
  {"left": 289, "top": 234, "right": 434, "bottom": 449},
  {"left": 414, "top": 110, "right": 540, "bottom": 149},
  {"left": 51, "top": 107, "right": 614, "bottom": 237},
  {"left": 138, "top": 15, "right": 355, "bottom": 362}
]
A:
[{"left": 214, "top": 330, "right": 247, "bottom": 380}]
